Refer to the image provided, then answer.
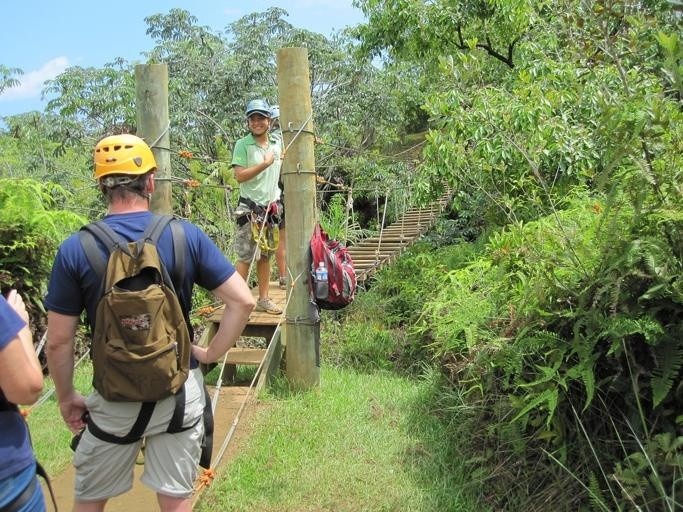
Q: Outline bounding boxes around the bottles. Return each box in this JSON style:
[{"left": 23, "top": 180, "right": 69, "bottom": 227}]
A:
[{"left": 314, "top": 261, "right": 331, "bottom": 299}]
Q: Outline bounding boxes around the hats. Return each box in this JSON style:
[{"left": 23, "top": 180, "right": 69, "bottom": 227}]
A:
[{"left": 247, "top": 111, "right": 270, "bottom": 118}]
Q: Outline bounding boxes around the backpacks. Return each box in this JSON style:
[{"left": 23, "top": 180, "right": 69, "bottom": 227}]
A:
[
  {"left": 80, "top": 215, "right": 192, "bottom": 401},
  {"left": 311, "top": 223, "right": 357, "bottom": 310}
]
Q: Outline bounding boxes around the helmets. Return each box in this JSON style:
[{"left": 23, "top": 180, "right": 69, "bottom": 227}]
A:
[
  {"left": 270, "top": 105, "right": 280, "bottom": 120},
  {"left": 93, "top": 134, "right": 158, "bottom": 180},
  {"left": 245, "top": 99, "right": 270, "bottom": 115}
]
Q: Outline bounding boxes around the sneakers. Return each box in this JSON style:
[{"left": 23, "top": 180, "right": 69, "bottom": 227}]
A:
[{"left": 256, "top": 297, "right": 283, "bottom": 315}]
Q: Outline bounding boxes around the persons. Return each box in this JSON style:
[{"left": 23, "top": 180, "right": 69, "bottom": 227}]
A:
[
  {"left": 265, "top": 106, "right": 289, "bottom": 292},
  {"left": 0, "top": 286, "right": 49, "bottom": 511},
  {"left": 232, "top": 99, "right": 289, "bottom": 318},
  {"left": 40, "top": 132, "right": 256, "bottom": 512}
]
